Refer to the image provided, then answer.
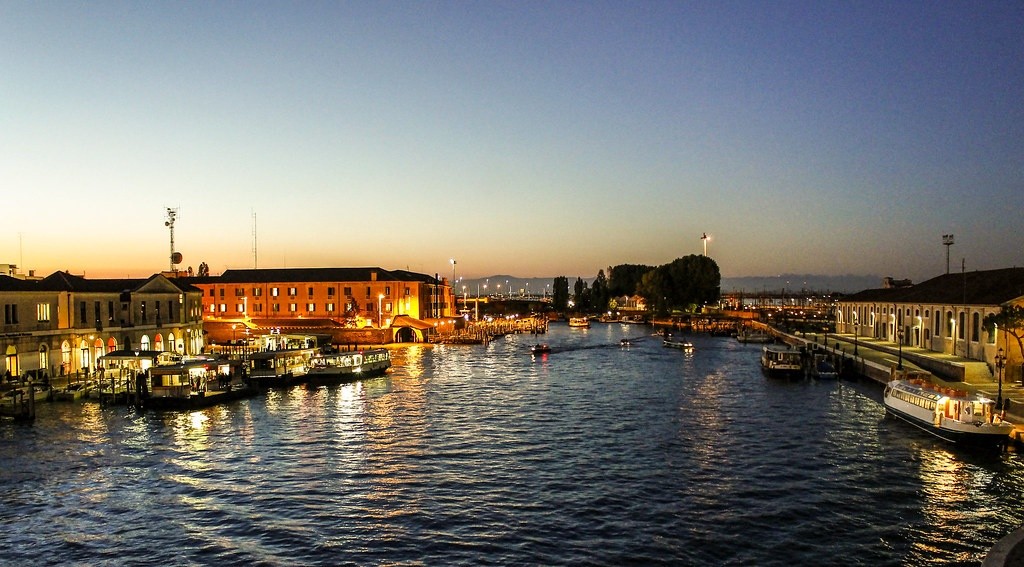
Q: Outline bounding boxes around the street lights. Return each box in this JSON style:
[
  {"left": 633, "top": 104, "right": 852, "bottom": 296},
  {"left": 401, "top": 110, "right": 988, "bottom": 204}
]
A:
[
  {"left": 701, "top": 232, "right": 712, "bottom": 256},
  {"left": 822, "top": 322, "right": 829, "bottom": 345},
  {"left": 994, "top": 347, "right": 1008, "bottom": 409},
  {"left": 853, "top": 319, "right": 860, "bottom": 355},
  {"left": 895, "top": 323, "right": 904, "bottom": 371},
  {"left": 378, "top": 294, "right": 385, "bottom": 328}
]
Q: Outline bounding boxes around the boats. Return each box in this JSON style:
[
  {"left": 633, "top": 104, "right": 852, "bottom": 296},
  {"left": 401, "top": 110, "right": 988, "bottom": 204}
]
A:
[
  {"left": 882, "top": 374, "right": 1016, "bottom": 445},
  {"left": 620, "top": 338, "right": 630, "bottom": 347},
  {"left": 530, "top": 343, "right": 551, "bottom": 352},
  {"left": 760, "top": 341, "right": 842, "bottom": 380},
  {"left": 708, "top": 317, "right": 777, "bottom": 344},
  {"left": 568, "top": 316, "right": 591, "bottom": 326},
  {"left": 598, "top": 311, "right": 645, "bottom": 324},
  {"left": 241, "top": 347, "right": 392, "bottom": 381},
  {"left": 0, "top": 379, "right": 134, "bottom": 405},
  {"left": 662, "top": 339, "right": 694, "bottom": 350}
]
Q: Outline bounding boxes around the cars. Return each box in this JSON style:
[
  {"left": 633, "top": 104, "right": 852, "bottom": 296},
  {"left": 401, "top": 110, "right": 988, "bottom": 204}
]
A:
[{"left": 483, "top": 314, "right": 493, "bottom": 320}]
